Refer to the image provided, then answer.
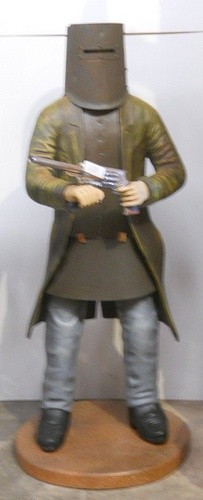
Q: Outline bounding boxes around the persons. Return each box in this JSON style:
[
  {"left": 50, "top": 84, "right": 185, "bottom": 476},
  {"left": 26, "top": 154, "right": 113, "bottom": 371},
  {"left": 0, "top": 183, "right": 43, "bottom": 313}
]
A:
[{"left": 26, "top": 21, "right": 188, "bottom": 452}]
